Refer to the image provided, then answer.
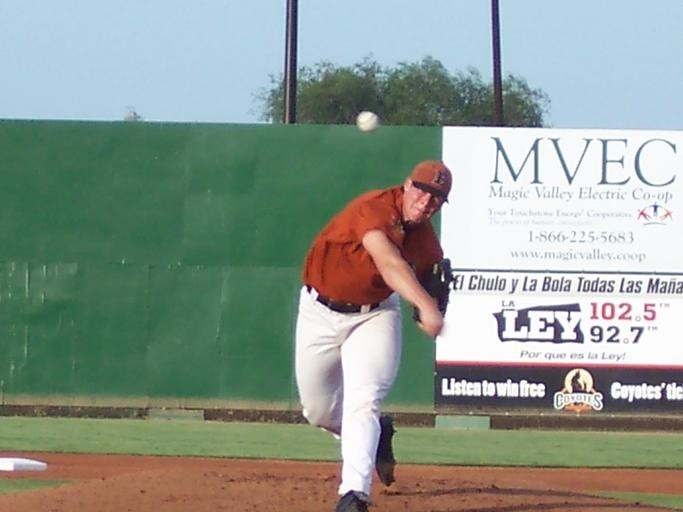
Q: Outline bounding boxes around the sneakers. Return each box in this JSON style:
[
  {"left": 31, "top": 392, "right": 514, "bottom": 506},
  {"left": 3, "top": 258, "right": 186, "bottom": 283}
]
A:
[
  {"left": 336, "top": 490, "right": 367, "bottom": 512},
  {"left": 375, "top": 416, "right": 396, "bottom": 486}
]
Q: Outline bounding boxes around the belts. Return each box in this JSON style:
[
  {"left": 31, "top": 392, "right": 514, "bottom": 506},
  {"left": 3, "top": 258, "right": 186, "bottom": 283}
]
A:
[{"left": 307, "top": 286, "right": 380, "bottom": 312}]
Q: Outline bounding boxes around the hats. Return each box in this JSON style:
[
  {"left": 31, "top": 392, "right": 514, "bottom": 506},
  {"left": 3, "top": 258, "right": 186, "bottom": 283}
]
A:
[{"left": 411, "top": 160, "right": 451, "bottom": 203}]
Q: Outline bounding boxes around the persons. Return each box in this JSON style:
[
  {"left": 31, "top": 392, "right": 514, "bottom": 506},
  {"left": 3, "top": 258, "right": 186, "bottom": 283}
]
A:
[{"left": 295, "top": 160, "right": 452, "bottom": 512}]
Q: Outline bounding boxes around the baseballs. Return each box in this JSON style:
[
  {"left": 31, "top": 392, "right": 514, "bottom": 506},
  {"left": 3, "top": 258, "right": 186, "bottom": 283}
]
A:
[{"left": 356, "top": 111, "right": 378, "bottom": 131}]
